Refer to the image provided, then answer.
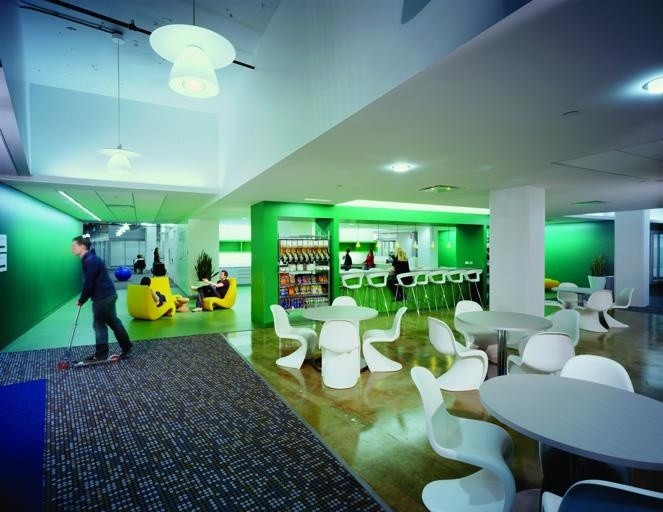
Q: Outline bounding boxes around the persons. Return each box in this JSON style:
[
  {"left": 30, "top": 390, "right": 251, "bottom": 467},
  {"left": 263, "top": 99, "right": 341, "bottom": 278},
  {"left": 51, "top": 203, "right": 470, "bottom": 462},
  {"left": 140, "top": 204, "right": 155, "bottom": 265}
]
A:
[
  {"left": 387, "top": 248, "right": 414, "bottom": 302},
  {"left": 153, "top": 248, "right": 160, "bottom": 264},
  {"left": 362, "top": 250, "right": 375, "bottom": 268},
  {"left": 344, "top": 249, "right": 352, "bottom": 270},
  {"left": 139, "top": 276, "right": 167, "bottom": 306},
  {"left": 71, "top": 235, "right": 134, "bottom": 361},
  {"left": 133, "top": 251, "right": 145, "bottom": 273},
  {"left": 189, "top": 269, "right": 230, "bottom": 312}
]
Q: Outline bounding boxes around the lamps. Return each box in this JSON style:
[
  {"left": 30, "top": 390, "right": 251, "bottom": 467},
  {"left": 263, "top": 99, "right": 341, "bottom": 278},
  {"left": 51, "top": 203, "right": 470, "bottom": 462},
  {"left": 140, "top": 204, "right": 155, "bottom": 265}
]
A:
[
  {"left": 98, "top": 33, "right": 140, "bottom": 177},
  {"left": 147, "top": 0, "right": 238, "bottom": 99},
  {"left": 355, "top": 220, "right": 453, "bottom": 249}
]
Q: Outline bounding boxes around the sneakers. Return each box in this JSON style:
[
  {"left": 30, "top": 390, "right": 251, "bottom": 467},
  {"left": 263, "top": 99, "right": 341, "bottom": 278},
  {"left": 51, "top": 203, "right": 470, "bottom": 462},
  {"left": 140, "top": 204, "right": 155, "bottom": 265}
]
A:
[
  {"left": 85, "top": 356, "right": 107, "bottom": 362},
  {"left": 191, "top": 286, "right": 198, "bottom": 290},
  {"left": 119, "top": 347, "right": 134, "bottom": 359},
  {"left": 192, "top": 307, "right": 203, "bottom": 311}
]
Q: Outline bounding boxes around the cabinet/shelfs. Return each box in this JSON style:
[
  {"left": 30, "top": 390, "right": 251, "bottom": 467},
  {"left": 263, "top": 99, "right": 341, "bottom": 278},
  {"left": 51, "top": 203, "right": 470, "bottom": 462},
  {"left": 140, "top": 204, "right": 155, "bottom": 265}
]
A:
[{"left": 277, "top": 229, "right": 331, "bottom": 324}]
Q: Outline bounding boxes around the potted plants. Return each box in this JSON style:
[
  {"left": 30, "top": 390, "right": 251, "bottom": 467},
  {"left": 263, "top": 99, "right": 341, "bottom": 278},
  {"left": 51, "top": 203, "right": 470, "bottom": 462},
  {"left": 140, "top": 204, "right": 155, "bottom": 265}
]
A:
[
  {"left": 586, "top": 256, "right": 608, "bottom": 289},
  {"left": 192, "top": 249, "right": 219, "bottom": 286}
]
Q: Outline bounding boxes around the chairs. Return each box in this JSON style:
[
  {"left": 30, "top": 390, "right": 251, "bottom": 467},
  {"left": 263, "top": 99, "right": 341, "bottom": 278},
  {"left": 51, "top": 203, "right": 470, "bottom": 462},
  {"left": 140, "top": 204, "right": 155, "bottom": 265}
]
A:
[
  {"left": 134, "top": 259, "right": 146, "bottom": 274},
  {"left": 200, "top": 276, "right": 238, "bottom": 312},
  {"left": 151, "top": 263, "right": 166, "bottom": 276},
  {"left": 127, "top": 283, "right": 177, "bottom": 320},
  {"left": 148, "top": 276, "right": 183, "bottom": 309}
]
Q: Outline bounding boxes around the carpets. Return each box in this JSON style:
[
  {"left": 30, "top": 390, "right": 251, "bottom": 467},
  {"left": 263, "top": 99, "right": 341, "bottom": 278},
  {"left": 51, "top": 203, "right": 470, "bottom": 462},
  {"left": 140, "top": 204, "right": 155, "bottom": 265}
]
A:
[
  {"left": 0, "top": 378, "right": 48, "bottom": 512},
  {"left": 0, "top": 333, "right": 392, "bottom": 512}
]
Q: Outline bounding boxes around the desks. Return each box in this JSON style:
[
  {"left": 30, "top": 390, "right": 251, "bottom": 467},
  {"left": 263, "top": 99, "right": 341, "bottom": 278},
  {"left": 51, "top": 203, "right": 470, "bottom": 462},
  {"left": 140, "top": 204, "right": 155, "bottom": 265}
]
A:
[
  {"left": 115, "top": 267, "right": 132, "bottom": 281},
  {"left": 177, "top": 298, "right": 189, "bottom": 312}
]
